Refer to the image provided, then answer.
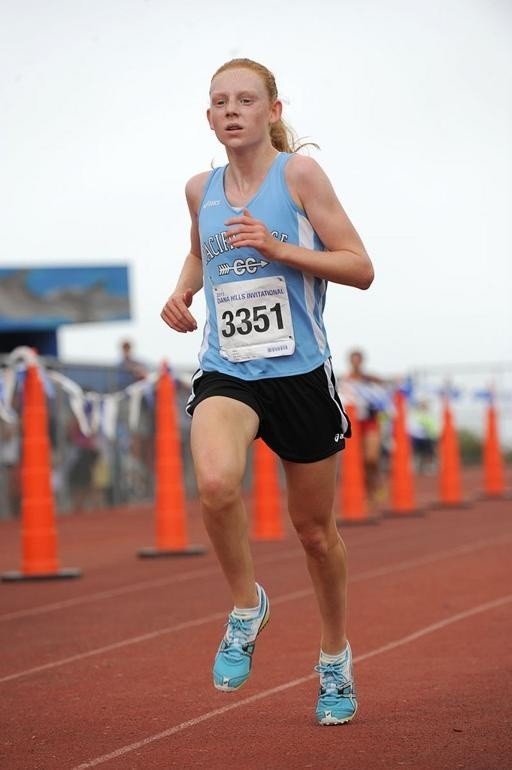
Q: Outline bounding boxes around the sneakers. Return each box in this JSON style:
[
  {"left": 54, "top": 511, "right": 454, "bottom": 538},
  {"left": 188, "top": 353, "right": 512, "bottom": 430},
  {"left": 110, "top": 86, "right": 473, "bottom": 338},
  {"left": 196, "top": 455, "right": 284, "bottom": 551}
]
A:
[
  {"left": 213, "top": 584, "right": 270, "bottom": 692},
  {"left": 314, "top": 642, "right": 358, "bottom": 727}
]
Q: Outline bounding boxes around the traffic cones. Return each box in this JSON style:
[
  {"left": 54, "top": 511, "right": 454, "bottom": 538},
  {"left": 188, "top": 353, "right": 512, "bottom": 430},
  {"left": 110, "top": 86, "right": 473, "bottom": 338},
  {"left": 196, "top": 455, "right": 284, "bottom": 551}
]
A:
[
  {"left": 4, "top": 366, "right": 86, "bottom": 585},
  {"left": 337, "top": 404, "right": 374, "bottom": 523},
  {"left": 435, "top": 402, "right": 472, "bottom": 508},
  {"left": 132, "top": 366, "right": 212, "bottom": 554},
  {"left": 478, "top": 405, "right": 512, "bottom": 498},
  {"left": 381, "top": 391, "right": 422, "bottom": 516},
  {"left": 249, "top": 440, "right": 290, "bottom": 536}
]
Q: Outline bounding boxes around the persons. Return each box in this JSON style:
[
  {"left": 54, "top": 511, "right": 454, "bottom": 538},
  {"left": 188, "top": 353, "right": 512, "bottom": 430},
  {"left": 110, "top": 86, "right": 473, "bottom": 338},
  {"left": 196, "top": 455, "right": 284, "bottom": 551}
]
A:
[
  {"left": 117, "top": 342, "right": 155, "bottom": 410},
  {"left": 344, "top": 353, "right": 390, "bottom": 491},
  {"left": 161, "top": 58, "right": 374, "bottom": 726}
]
